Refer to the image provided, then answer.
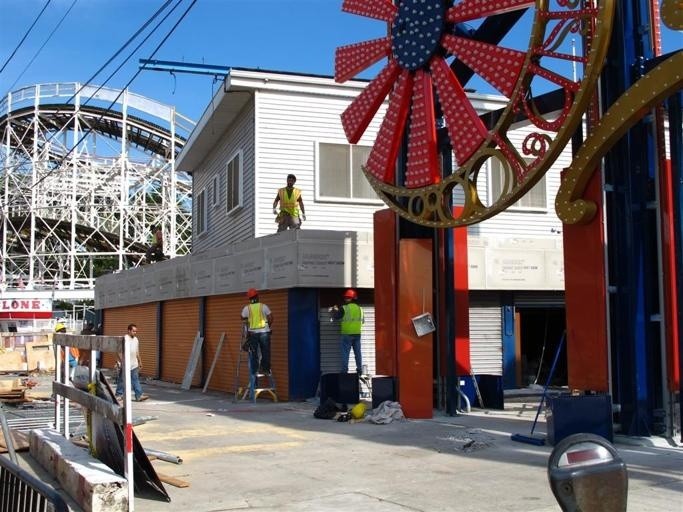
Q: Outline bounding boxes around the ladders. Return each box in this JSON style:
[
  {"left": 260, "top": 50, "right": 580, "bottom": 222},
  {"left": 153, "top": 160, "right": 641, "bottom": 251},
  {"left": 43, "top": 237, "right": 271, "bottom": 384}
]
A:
[{"left": 235, "top": 320, "right": 280, "bottom": 403}]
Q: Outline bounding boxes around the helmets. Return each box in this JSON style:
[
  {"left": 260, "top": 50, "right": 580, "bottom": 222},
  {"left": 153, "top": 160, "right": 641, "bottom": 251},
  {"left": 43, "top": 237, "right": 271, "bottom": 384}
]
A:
[
  {"left": 351, "top": 403, "right": 366, "bottom": 420},
  {"left": 247, "top": 288, "right": 257, "bottom": 297},
  {"left": 343, "top": 289, "right": 358, "bottom": 299}
]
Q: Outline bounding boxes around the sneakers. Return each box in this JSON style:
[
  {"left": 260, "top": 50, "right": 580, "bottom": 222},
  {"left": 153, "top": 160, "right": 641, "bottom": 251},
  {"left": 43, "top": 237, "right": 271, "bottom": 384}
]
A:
[
  {"left": 136, "top": 395, "right": 149, "bottom": 402},
  {"left": 116, "top": 396, "right": 122, "bottom": 401}
]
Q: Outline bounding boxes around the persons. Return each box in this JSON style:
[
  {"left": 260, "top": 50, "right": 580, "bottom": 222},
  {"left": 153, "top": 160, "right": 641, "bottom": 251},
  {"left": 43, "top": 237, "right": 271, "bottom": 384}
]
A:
[
  {"left": 140, "top": 224, "right": 170, "bottom": 265},
  {"left": 55, "top": 324, "right": 100, "bottom": 383},
  {"left": 273, "top": 174, "right": 306, "bottom": 233},
  {"left": 115, "top": 324, "right": 149, "bottom": 402},
  {"left": 329, "top": 289, "right": 364, "bottom": 375},
  {"left": 240, "top": 288, "right": 275, "bottom": 377}
]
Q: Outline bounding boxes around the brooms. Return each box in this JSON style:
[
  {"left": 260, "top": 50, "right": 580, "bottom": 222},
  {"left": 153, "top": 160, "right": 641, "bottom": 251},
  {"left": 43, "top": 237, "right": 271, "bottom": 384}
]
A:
[{"left": 510, "top": 330, "right": 567, "bottom": 446}]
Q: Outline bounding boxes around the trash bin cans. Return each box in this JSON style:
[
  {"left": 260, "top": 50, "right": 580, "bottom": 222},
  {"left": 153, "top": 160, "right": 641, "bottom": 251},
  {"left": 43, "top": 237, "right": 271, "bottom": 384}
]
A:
[{"left": 372, "top": 375, "right": 397, "bottom": 410}]
